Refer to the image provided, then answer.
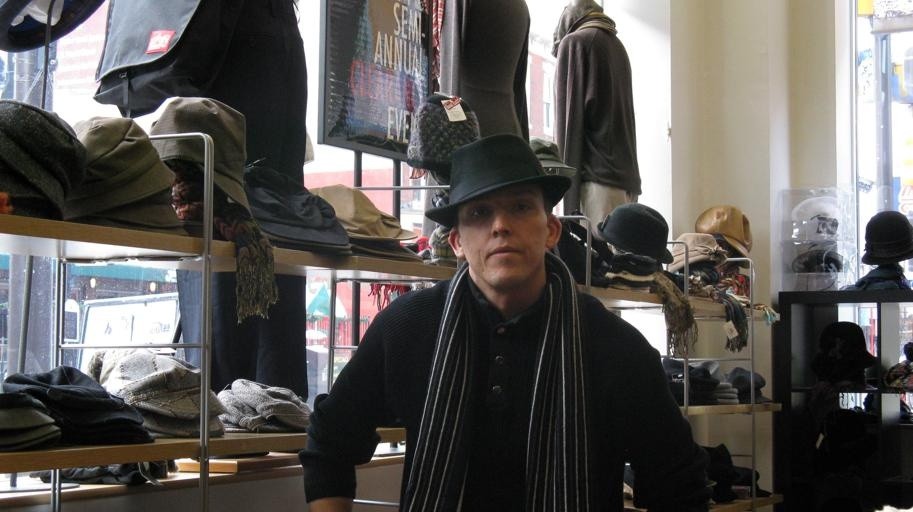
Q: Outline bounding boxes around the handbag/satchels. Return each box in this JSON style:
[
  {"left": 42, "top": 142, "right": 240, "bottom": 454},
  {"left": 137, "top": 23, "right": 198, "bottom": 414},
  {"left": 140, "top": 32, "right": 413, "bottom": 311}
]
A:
[{"left": 94, "top": 0, "right": 241, "bottom": 113}]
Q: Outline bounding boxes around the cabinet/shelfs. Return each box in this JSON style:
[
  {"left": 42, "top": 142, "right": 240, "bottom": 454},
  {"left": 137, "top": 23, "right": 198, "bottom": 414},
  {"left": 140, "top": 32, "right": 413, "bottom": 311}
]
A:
[
  {"left": 772, "top": 289, "right": 913, "bottom": 512},
  {"left": 0, "top": 131, "right": 780, "bottom": 512}
]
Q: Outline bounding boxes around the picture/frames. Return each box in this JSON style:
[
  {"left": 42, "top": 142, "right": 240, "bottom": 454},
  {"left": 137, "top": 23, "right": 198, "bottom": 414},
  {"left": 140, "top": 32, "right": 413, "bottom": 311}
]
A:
[{"left": 316, "top": 0, "right": 461, "bottom": 173}]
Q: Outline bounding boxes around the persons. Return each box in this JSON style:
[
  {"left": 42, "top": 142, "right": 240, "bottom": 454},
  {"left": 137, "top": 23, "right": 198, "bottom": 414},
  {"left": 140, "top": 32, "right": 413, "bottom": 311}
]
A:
[
  {"left": 552, "top": 1, "right": 642, "bottom": 236},
  {"left": 437, "top": 1, "right": 530, "bottom": 148},
  {"left": 301, "top": 134, "right": 705, "bottom": 511}
]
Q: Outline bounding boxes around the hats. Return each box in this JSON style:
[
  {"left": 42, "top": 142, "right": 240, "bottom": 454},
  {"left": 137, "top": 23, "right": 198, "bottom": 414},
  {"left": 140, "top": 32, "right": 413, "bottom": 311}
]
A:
[
  {"left": 217, "top": 413, "right": 248, "bottom": 433},
  {"left": 91, "top": 188, "right": 187, "bottom": 236},
  {"left": 702, "top": 444, "right": 759, "bottom": 485},
  {"left": 60, "top": 116, "right": 173, "bottom": 219},
  {"left": 102, "top": 349, "right": 226, "bottom": 419},
  {"left": 810, "top": 322, "right": 877, "bottom": 373},
  {"left": 2, "top": 366, "right": 155, "bottom": 443},
  {"left": 598, "top": 204, "right": 673, "bottom": 266},
  {"left": 0, "top": 392, "right": 60, "bottom": 451},
  {"left": 147, "top": 98, "right": 252, "bottom": 217},
  {"left": 696, "top": 205, "right": 753, "bottom": 268},
  {"left": 406, "top": 95, "right": 480, "bottom": 168},
  {"left": 232, "top": 378, "right": 314, "bottom": 429},
  {"left": 862, "top": 212, "right": 913, "bottom": 264},
  {"left": 0, "top": 159, "right": 55, "bottom": 219},
  {"left": 0, "top": 100, "right": 87, "bottom": 214},
  {"left": 790, "top": 197, "right": 842, "bottom": 243},
  {"left": 308, "top": 185, "right": 422, "bottom": 262},
  {"left": 791, "top": 251, "right": 847, "bottom": 275},
  {"left": 528, "top": 137, "right": 576, "bottom": 179},
  {"left": 1, "top": 0, "right": 103, "bottom": 53},
  {"left": 667, "top": 232, "right": 726, "bottom": 274},
  {"left": 426, "top": 133, "right": 572, "bottom": 228},
  {"left": 242, "top": 170, "right": 352, "bottom": 257},
  {"left": 137, "top": 408, "right": 224, "bottom": 438},
  {"left": 817, "top": 413, "right": 873, "bottom": 460},
  {"left": 219, "top": 391, "right": 291, "bottom": 433}
]
